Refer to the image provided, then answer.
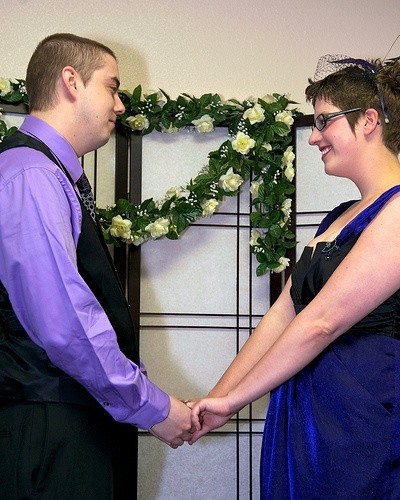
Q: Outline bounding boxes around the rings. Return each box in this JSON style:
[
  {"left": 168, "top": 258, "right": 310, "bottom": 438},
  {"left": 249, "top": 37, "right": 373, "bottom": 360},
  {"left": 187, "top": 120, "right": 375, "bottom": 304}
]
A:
[{"left": 184, "top": 399, "right": 192, "bottom": 404}]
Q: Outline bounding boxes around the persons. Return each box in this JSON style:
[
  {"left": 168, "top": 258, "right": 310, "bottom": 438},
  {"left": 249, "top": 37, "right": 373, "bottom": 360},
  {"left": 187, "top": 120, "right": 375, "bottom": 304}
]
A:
[
  {"left": 0, "top": 33, "right": 195, "bottom": 500},
  {"left": 185, "top": 55, "right": 400, "bottom": 500}
]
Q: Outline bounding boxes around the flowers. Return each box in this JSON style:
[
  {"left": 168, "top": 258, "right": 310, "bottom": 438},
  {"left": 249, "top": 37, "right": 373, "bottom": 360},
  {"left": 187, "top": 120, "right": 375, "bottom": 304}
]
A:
[{"left": 0, "top": 77, "right": 304, "bottom": 277}]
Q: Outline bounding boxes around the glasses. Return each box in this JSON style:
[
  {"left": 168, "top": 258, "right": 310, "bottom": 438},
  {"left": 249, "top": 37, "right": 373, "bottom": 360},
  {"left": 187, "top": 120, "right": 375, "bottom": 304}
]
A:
[{"left": 311, "top": 107, "right": 380, "bottom": 133}]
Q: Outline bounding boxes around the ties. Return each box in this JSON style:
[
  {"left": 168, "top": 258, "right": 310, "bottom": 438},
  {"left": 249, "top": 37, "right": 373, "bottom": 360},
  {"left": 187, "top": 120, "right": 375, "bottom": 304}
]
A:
[{"left": 77, "top": 172, "right": 97, "bottom": 225}]
